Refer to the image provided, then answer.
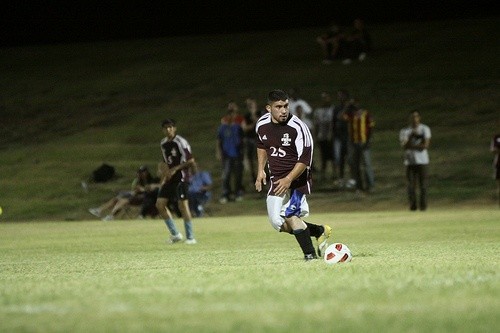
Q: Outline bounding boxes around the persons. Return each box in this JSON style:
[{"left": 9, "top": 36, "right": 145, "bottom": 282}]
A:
[
  {"left": 87, "top": 156, "right": 212, "bottom": 220},
  {"left": 253, "top": 90, "right": 332, "bottom": 260},
  {"left": 398, "top": 109, "right": 432, "bottom": 212},
  {"left": 218, "top": 88, "right": 358, "bottom": 193},
  {"left": 155, "top": 117, "right": 198, "bottom": 245},
  {"left": 337, "top": 95, "right": 376, "bottom": 193},
  {"left": 490, "top": 127, "right": 500, "bottom": 199},
  {"left": 217, "top": 109, "right": 246, "bottom": 203}
]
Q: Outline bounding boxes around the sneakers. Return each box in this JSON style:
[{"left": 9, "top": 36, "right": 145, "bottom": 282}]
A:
[
  {"left": 316, "top": 224, "right": 332, "bottom": 257},
  {"left": 169, "top": 232, "right": 182, "bottom": 244},
  {"left": 185, "top": 238, "right": 196, "bottom": 245},
  {"left": 89, "top": 209, "right": 101, "bottom": 217},
  {"left": 103, "top": 215, "right": 114, "bottom": 221}
]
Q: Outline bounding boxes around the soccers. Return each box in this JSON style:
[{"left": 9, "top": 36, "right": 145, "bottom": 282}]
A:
[{"left": 324, "top": 243, "right": 352, "bottom": 264}]
